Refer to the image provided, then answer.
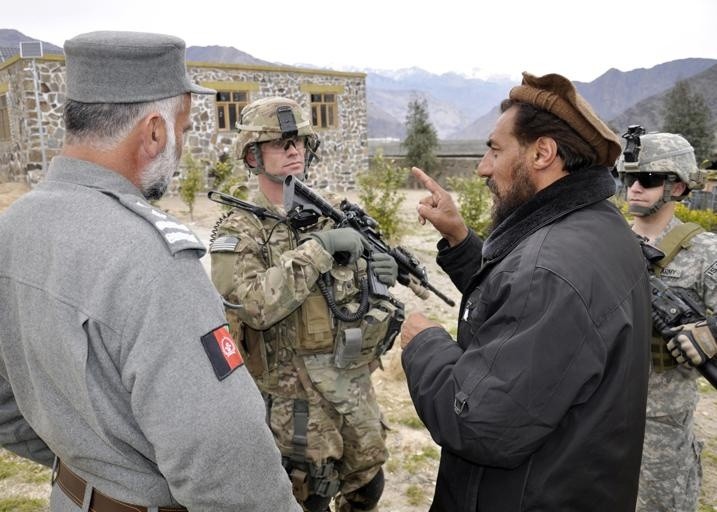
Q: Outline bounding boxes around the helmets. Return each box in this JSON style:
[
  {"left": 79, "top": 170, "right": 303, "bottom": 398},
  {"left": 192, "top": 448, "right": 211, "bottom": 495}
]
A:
[{"left": 617, "top": 132, "right": 706, "bottom": 189}]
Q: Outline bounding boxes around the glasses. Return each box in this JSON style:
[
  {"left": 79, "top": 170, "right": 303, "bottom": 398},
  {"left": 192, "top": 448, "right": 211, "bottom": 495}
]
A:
[
  {"left": 623, "top": 172, "right": 668, "bottom": 189},
  {"left": 261, "top": 136, "right": 305, "bottom": 153}
]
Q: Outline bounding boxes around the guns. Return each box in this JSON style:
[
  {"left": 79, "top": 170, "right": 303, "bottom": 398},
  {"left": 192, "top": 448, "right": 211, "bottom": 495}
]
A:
[
  {"left": 282, "top": 175, "right": 456, "bottom": 306},
  {"left": 639, "top": 237, "right": 715, "bottom": 384}
]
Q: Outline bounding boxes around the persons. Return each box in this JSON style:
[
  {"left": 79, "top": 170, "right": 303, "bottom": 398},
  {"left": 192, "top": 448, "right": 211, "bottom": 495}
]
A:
[
  {"left": 400, "top": 73, "right": 653, "bottom": 512},
  {"left": 207, "top": 97, "right": 405, "bottom": 511},
  {"left": 610, "top": 125, "right": 715, "bottom": 512},
  {"left": 0, "top": 28, "right": 305, "bottom": 510}
]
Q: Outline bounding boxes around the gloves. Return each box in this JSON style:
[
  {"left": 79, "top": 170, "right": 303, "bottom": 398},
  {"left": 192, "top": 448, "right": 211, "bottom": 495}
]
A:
[
  {"left": 371, "top": 253, "right": 398, "bottom": 286},
  {"left": 311, "top": 227, "right": 373, "bottom": 263},
  {"left": 661, "top": 317, "right": 717, "bottom": 369}
]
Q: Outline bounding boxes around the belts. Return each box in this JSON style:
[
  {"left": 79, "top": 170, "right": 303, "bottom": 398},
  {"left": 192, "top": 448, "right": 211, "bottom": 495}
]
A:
[{"left": 55, "top": 460, "right": 188, "bottom": 512}]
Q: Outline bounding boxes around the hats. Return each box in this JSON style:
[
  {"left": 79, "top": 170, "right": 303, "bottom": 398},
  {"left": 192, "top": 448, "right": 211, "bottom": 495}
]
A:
[
  {"left": 234, "top": 96, "right": 316, "bottom": 160},
  {"left": 509, "top": 70, "right": 622, "bottom": 167},
  {"left": 64, "top": 30, "right": 218, "bottom": 104}
]
